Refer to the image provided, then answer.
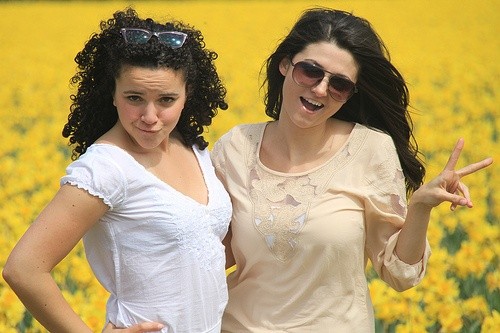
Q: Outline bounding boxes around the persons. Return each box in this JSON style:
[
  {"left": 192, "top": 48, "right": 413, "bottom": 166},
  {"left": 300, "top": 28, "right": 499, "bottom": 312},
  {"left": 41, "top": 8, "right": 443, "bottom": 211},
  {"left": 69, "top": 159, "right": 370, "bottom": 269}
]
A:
[
  {"left": 2, "top": 5, "right": 233, "bottom": 333},
  {"left": 209, "top": 2, "right": 495, "bottom": 333}
]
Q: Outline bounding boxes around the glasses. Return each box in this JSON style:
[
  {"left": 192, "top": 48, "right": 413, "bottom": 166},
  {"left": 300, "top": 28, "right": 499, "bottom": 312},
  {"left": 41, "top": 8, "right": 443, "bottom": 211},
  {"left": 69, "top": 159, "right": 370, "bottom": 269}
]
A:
[
  {"left": 120, "top": 28, "right": 188, "bottom": 49},
  {"left": 289, "top": 60, "right": 357, "bottom": 102}
]
